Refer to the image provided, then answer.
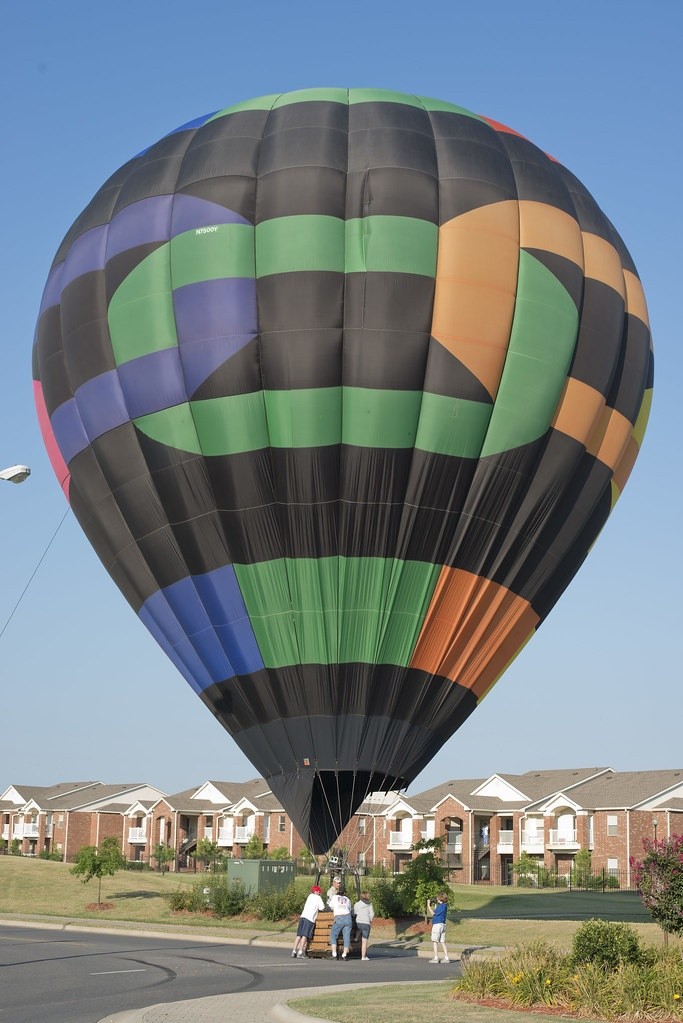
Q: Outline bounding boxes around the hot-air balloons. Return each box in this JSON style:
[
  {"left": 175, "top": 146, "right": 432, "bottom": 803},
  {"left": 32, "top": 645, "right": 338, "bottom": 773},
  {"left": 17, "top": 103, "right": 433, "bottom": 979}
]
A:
[{"left": 33, "top": 88, "right": 655, "bottom": 961}]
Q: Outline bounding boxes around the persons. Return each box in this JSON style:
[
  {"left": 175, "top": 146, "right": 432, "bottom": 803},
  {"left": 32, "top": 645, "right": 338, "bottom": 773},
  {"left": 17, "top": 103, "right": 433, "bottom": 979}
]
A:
[
  {"left": 291, "top": 886, "right": 326, "bottom": 960},
  {"left": 428, "top": 892, "right": 451, "bottom": 964},
  {"left": 330, "top": 886, "right": 353, "bottom": 961},
  {"left": 326, "top": 877, "right": 341, "bottom": 910},
  {"left": 353, "top": 890, "right": 374, "bottom": 961}
]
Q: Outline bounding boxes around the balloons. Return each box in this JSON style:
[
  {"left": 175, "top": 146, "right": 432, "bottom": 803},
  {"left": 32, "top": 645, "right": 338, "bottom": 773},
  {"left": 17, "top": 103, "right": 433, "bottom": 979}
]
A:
[{"left": 34, "top": 88, "right": 655, "bottom": 856}]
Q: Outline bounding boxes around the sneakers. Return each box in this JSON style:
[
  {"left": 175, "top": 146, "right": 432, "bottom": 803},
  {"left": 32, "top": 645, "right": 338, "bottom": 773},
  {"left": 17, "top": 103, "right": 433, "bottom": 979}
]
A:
[
  {"left": 362, "top": 957, "right": 370, "bottom": 961},
  {"left": 440, "top": 958, "right": 450, "bottom": 964},
  {"left": 339, "top": 955, "right": 346, "bottom": 960},
  {"left": 291, "top": 951, "right": 309, "bottom": 958},
  {"left": 326, "top": 956, "right": 338, "bottom": 961},
  {"left": 430, "top": 957, "right": 439, "bottom": 963}
]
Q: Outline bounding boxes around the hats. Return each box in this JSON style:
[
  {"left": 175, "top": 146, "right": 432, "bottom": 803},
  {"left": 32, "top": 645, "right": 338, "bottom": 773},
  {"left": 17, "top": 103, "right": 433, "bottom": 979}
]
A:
[
  {"left": 311, "top": 885, "right": 323, "bottom": 892},
  {"left": 333, "top": 877, "right": 342, "bottom": 882},
  {"left": 339, "top": 887, "right": 347, "bottom": 892}
]
